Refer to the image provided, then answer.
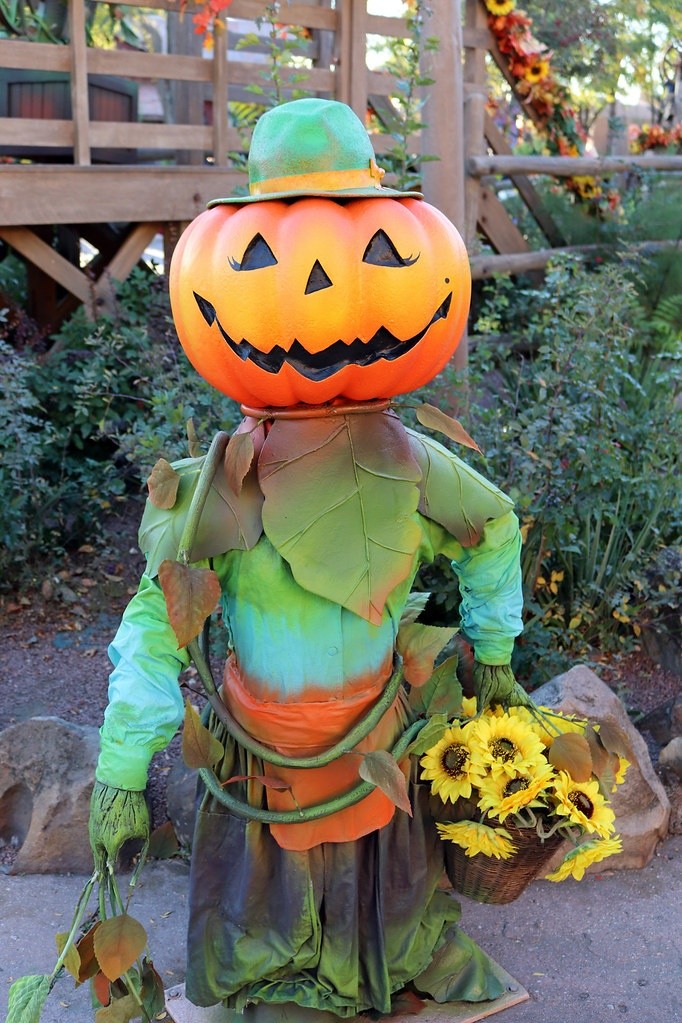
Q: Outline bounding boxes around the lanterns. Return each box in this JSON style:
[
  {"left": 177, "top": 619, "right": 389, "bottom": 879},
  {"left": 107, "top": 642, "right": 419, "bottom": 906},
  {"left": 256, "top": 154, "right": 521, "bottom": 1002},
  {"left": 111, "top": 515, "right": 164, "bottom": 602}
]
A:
[{"left": 167, "top": 198, "right": 473, "bottom": 408}]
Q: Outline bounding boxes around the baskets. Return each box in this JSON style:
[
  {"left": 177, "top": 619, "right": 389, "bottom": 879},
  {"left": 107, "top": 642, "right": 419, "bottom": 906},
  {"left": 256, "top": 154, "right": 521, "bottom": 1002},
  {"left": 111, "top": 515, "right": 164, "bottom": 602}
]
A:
[{"left": 443, "top": 792, "right": 577, "bottom": 903}]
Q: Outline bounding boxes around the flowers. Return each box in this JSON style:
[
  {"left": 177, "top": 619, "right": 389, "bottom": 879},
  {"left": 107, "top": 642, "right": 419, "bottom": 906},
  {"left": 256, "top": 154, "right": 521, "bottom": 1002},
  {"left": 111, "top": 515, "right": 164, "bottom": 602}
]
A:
[{"left": 415, "top": 695, "right": 632, "bottom": 882}]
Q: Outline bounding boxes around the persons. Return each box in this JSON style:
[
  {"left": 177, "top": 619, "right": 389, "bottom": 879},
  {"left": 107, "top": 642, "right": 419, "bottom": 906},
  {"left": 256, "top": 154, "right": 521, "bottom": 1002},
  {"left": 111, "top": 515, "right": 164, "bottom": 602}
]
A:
[
  {"left": 629, "top": 132, "right": 656, "bottom": 191},
  {"left": 578, "top": 123, "right": 601, "bottom": 158}
]
